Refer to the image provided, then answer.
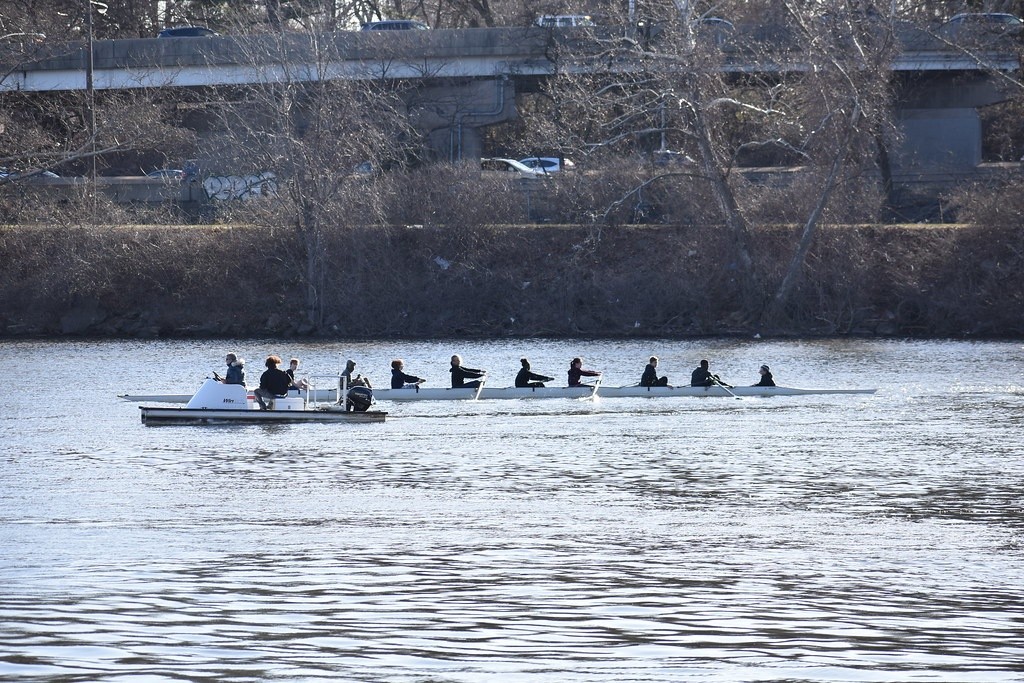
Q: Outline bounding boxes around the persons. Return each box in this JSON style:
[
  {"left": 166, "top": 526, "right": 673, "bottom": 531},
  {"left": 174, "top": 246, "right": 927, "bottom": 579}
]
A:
[
  {"left": 391, "top": 360, "right": 423, "bottom": 389},
  {"left": 449, "top": 355, "right": 486, "bottom": 388},
  {"left": 641, "top": 356, "right": 668, "bottom": 387},
  {"left": 221, "top": 352, "right": 246, "bottom": 387},
  {"left": 691, "top": 359, "right": 720, "bottom": 387},
  {"left": 568, "top": 358, "right": 601, "bottom": 387},
  {"left": 286, "top": 358, "right": 315, "bottom": 394},
  {"left": 750, "top": 364, "right": 775, "bottom": 386},
  {"left": 515, "top": 358, "right": 555, "bottom": 388},
  {"left": 254, "top": 356, "right": 291, "bottom": 410},
  {"left": 340, "top": 360, "right": 365, "bottom": 389}
]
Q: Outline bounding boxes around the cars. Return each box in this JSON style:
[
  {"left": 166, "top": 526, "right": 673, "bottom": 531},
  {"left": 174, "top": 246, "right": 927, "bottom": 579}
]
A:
[
  {"left": 520, "top": 155, "right": 576, "bottom": 173},
  {"left": 359, "top": 19, "right": 429, "bottom": 31},
  {"left": 478, "top": 159, "right": 553, "bottom": 180},
  {"left": 637, "top": 151, "right": 698, "bottom": 169},
  {"left": 0, "top": 166, "right": 60, "bottom": 179},
  {"left": 156, "top": 24, "right": 222, "bottom": 38},
  {"left": 0, "top": 32, "right": 48, "bottom": 45},
  {"left": 531, "top": 14, "right": 596, "bottom": 26},
  {"left": 146, "top": 168, "right": 184, "bottom": 177},
  {"left": 686, "top": 17, "right": 739, "bottom": 53}
]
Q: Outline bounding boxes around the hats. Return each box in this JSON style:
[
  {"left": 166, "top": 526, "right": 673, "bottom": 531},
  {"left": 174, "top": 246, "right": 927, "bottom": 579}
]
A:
[
  {"left": 347, "top": 360, "right": 356, "bottom": 366},
  {"left": 762, "top": 365, "right": 769, "bottom": 371}
]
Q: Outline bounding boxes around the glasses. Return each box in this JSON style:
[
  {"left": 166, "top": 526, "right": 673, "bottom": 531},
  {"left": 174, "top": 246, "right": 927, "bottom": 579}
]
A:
[{"left": 226, "top": 358, "right": 228, "bottom": 359}]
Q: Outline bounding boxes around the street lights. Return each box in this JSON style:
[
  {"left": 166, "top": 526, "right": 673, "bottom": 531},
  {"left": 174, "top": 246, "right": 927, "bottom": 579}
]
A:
[{"left": 83, "top": 0, "right": 109, "bottom": 223}]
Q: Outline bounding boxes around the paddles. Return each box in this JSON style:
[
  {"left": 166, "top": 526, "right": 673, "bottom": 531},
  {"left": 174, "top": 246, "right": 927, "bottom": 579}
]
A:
[
  {"left": 472, "top": 370, "right": 487, "bottom": 404},
  {"left": 585, "top": 370, "right": 604, "bottom": 407},
  {"left": 357, "top": 373, "right": 379, "bottom": 411},
  {"left": 709, "top": 374, "right": 742, "bottom": 403}
]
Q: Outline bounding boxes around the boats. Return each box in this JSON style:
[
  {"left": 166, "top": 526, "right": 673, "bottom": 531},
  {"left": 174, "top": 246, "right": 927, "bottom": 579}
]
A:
[
  {"left": 116, "top": 384, "right": 882, "bottom": 402},
  {"left": 135, "top": 373, "right": 391, "bottom": 427}
]
